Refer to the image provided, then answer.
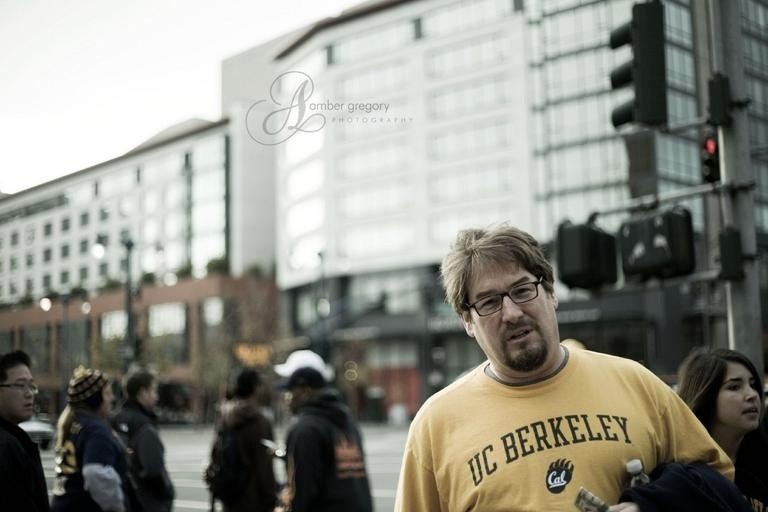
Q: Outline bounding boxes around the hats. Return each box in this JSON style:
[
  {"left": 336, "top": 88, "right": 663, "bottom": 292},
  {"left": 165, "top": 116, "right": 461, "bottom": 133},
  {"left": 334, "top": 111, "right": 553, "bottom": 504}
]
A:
[
  {"left": 67, "top": 367, "right": 109, "bottom": 407},
  {"left": 272, "top": 350, "right": 329, "bottom": 390}
]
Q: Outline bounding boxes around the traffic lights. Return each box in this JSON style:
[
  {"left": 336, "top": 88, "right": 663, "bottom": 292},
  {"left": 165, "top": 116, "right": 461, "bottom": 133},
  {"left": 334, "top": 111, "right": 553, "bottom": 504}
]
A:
[
  {"left": 698, "top": 133, "right": 719, "bottom": 183},
  {"left": 612, "top": 2, "right": 668, "bottom": 130}
]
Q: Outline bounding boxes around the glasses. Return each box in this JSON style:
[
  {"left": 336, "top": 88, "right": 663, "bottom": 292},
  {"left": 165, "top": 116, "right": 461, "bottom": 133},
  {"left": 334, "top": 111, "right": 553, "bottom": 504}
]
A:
[
  {"left": 465, "top": 275, "right": 543, "bottom": 316},
  {"left": 1, "top": 384, "right": 40, "bottom": 394}
]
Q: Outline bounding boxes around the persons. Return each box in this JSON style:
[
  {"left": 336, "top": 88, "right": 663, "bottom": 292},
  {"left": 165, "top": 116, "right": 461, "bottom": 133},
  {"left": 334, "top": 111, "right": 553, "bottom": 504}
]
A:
[
  {"left": 395, "top": 221, "right": 737, "bottom": 512},
  {"left": 268, "top": 369, "right": 374, "bottom": 512},
  {"left": 204, "top": 368, "right": 281, "bottom": 510},
  {"left": 45, "top": 366, "right": 143, "bottom": 511},
  {"left": 1, "top": 349, "right": 51, "bottom": 512},
  {"left": 677, "top": 342, "right": 768, "bottom": 510},
  {"left": 115, "top": 368, "right": 176, "bottom": 511}
]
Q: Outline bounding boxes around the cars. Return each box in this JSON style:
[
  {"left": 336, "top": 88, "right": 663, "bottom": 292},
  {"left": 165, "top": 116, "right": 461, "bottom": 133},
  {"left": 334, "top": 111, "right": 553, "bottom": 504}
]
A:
[{"left": 22, "top": 411, "right": 54, "bottom": 450}]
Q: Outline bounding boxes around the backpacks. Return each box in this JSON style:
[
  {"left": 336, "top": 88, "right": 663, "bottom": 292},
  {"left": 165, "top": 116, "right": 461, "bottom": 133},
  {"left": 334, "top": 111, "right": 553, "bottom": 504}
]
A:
[{"left": 207, "top": 419, "right": 253, "bottom": 501}]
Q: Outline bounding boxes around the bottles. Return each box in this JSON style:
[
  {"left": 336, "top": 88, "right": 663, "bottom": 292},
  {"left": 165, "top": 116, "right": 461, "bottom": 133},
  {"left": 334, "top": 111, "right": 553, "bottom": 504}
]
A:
[{"left": 625, "top": 457, "right": 651, "bottom": 490}]
